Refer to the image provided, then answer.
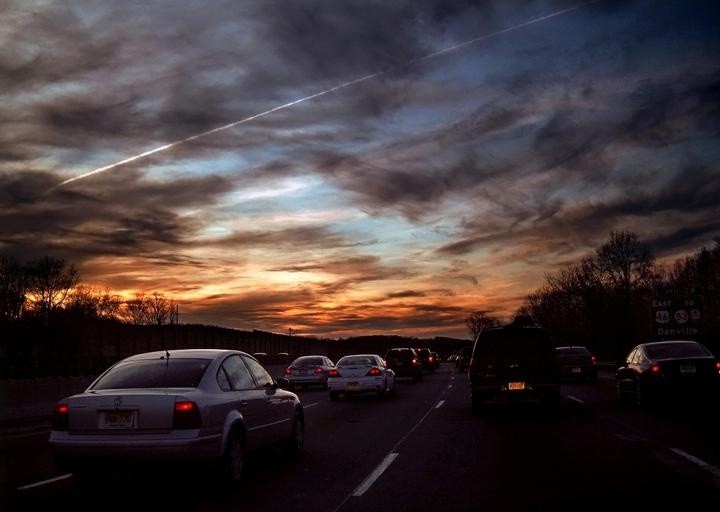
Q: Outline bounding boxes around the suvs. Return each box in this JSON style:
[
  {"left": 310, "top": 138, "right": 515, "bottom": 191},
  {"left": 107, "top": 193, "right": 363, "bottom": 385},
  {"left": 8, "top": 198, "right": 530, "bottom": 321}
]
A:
[
  {"left": 384, "top": 346, "right": 436, "bottom": 381},
  {"left": 467, "top": 324, "right": 563, "bottom": 409}
]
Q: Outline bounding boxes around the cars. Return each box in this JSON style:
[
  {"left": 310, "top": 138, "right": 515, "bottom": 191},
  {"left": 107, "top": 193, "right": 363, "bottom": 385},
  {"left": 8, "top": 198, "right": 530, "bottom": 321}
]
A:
[
  {"left": 285, "top": 354, "right": 396, "bottom": 400},
  {"left": 614, "top": 338, "right": 720, "bottom": 406},
  {"left": 555, "top": 345, "right": 600, "bottom": 383},
  {"left": 50, "top": 346, "right": 307, "bottom": 482}
]
